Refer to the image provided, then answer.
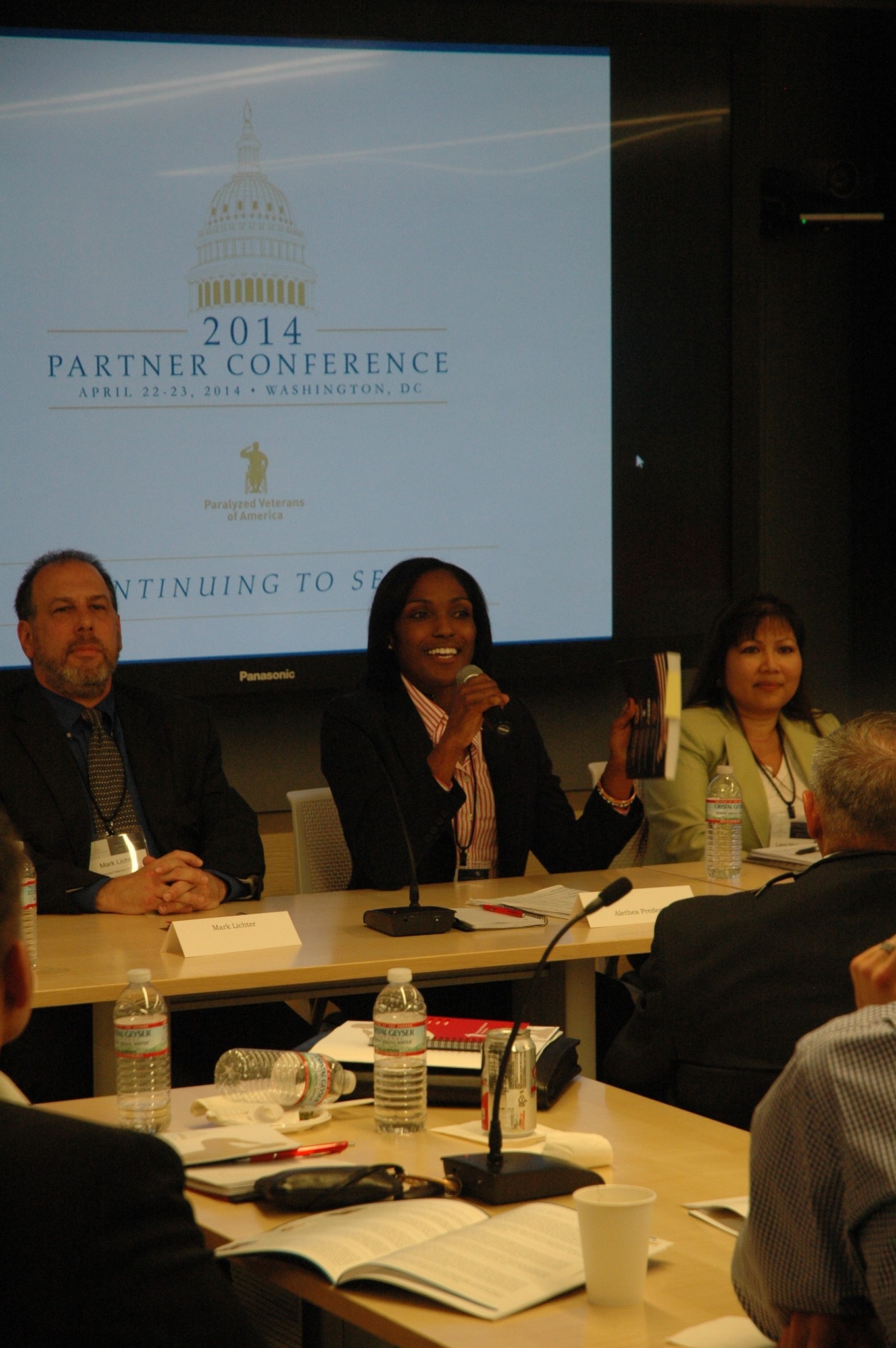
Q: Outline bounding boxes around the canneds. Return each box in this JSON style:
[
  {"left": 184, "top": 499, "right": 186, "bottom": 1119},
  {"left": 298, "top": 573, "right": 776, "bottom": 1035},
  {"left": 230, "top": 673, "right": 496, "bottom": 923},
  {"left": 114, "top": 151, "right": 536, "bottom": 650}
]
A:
[{"left": 479, "top": 1028, "right": 537, "bottom": 1137}]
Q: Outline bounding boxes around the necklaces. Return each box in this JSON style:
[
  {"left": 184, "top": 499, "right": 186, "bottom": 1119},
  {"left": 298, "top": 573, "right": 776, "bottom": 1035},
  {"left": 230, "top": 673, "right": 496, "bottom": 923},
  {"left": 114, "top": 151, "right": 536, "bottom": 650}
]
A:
[
  {"left": 732, "top": 705, "right": 805, "bottom": 818},
  {"left": 454, "top": 740, "right": 479, "bottom": 865}
]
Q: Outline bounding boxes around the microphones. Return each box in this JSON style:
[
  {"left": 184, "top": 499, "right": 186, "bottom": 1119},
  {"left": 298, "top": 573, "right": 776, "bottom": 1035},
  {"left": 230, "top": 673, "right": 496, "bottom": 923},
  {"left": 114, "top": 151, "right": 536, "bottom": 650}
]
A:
[
  {"left": 457, "top": 664, "right": 510, "bottom": 735},
  {"left": 442, "top": 875, "right": 633, "bottom": 1207}
]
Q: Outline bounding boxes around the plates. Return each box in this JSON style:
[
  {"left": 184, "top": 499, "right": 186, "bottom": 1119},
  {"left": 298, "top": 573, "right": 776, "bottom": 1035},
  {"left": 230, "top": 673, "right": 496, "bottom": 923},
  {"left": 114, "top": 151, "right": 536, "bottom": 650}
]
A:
[{"left": 220, "top": 1109, "right": 333, "bottom": 1133}]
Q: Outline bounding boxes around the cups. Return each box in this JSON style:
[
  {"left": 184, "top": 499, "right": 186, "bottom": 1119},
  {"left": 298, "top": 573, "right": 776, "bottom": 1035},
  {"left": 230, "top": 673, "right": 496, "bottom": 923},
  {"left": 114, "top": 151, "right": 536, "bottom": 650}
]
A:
[{"left": 572, "top": 1184, "right": 658, "bottom": 1308}]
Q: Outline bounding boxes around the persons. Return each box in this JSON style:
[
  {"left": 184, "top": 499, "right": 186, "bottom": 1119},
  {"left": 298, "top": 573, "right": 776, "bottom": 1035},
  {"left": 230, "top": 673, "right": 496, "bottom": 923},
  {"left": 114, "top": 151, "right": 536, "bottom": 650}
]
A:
[
  {"left": 749, "top": 937, "right": 896, "bottom": 1206},
  {"left": 0, "top": 550, "right": 318, "bottom": 1105},
  {"left": 320, "top": 559, "right": 645, "bottom": 1064},
  {"left": 596, "top": 711, "right": 896, "bottom": 1130},
  {"left": 643, "top": 591, "right": 839, "bottom": 864},
  {"left": 0, "top": 802, "right": 253, "bottom": 1348}
]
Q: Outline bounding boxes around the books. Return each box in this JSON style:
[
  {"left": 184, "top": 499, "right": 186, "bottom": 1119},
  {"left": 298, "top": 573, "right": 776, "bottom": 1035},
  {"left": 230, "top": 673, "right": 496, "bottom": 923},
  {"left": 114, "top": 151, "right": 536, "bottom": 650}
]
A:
[
  {"left": 450, "top": 902, "right": 549, "bottom": 932},
  {"left": 287, "top": 1016, "right": 583, "bottom": 1110},
  {"left": 155, "top": 1122, "right": 359, "bottom": 1205},
  {"left": 742, "top": 842, "right": 823, "bottom": 872},
  {"left": 215, "top": 1196, "right": 669, "bottom": 1323},
  {"left": 625, "top": 652, "right": 683, "bottom": 782},
  {"left": 681, "top": 1194, "right": 750, "bottom": 1238},
  {"left": 215, "top": 1198, "right": 676, "bottom": 1322}
]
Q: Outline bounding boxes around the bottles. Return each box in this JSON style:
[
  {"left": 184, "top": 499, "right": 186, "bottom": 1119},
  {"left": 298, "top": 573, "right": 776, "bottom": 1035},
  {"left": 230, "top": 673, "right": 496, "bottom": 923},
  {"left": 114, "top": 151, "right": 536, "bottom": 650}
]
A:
[
  {"left": 372, "top": 967, "right": 428, "bottom": 1136},
  {"left": 214, "top": 1047, "right": 357, "bottom": 1110},
  {"left": 12, "top": 841, "right": 37, "bottom": 970},
  {"left": 113, "top": 968, "right": 172, "bottom": 1134},
  {"left": 705, "top": 764, "right": 743, "bottom": 880}
]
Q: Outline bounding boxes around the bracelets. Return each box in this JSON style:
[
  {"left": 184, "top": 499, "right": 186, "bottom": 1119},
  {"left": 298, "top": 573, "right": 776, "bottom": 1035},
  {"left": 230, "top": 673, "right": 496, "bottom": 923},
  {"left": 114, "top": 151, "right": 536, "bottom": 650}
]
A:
[{"left": 597, "top": 777, "right": 636, "bottom": 807}]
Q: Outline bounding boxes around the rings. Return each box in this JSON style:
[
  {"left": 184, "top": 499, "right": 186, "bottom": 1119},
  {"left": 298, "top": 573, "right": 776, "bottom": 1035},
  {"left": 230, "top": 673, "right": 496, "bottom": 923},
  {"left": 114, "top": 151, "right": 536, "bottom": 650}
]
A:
[{"left": 880, "top": 941, "right": 895, "bottom": 954}]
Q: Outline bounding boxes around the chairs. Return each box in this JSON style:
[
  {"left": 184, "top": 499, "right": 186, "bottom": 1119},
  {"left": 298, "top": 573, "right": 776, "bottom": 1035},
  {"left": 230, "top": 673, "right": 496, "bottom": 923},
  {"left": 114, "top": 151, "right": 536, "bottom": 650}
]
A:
[
  {"left": 588, "top": 759, "right": 656, "bottom": 989},
  {"left": 285, "top": 788, "right": 363, "bottom": 1030}
]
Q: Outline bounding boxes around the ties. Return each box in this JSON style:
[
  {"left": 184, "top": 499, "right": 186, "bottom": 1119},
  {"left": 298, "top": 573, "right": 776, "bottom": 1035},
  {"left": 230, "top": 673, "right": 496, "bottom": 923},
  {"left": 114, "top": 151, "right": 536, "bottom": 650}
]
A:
[{"left": 82, "top": 706, "right": 137, "bottom": 836}]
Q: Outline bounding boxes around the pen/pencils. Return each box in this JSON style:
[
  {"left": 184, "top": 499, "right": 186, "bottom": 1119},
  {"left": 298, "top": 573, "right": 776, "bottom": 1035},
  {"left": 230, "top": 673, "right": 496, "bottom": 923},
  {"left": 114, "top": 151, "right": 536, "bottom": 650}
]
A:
[
  {"left": 795, "top": 845, "right": 820, "bottom": 855},
  {"left": 235, "top": 1140, "right": 354, "bottom": 1164},
  {"left": 482, "top": 903, "right": 525, "bottom": 919}
]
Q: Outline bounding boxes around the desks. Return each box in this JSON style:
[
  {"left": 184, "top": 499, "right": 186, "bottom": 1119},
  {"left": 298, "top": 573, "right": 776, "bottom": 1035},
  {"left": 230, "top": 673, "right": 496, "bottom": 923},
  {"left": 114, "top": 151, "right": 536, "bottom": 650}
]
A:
[
  {"left": 27, "top": 1077, "right": 777, "bottom": 1348},
  {"left": 27, "top": 843, "right": 842, "bottom": 1099}
]
